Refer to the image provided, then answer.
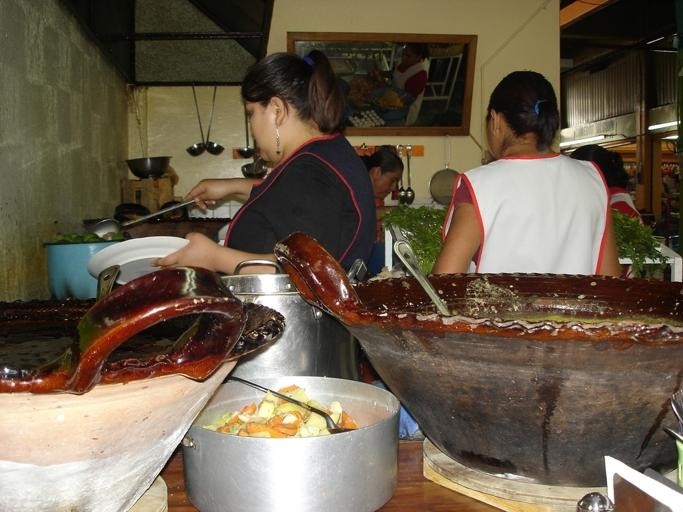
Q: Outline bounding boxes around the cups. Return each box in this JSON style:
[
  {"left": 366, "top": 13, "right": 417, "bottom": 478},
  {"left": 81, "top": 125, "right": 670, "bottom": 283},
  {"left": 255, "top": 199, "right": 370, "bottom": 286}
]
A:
[{"left": 650, "top": 236, "right": 667, "bottom": 254}]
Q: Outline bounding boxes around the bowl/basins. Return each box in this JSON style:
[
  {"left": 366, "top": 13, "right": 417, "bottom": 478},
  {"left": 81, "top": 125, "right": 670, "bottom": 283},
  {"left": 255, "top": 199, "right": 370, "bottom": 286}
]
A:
[
  {"left": 125, "top": 156, "right": 172, "bottom": 179},
  {"left": 120, "top": 218, "right": 230, "bottom": 242},
  {"left": 0, "top": 267, "right": 285, "bottom": 512},
  {"left": 276, "top": 231, "right": 683, "bottom": 488}
]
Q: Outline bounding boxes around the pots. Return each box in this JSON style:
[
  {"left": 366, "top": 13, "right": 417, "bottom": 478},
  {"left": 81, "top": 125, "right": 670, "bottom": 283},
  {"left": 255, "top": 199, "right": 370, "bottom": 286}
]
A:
[
  {"left": 184, "top": 376, "right": 399, "bottom": 511},
  {"left": 41, "top": 235, "right": 123, "bottom": 300}
]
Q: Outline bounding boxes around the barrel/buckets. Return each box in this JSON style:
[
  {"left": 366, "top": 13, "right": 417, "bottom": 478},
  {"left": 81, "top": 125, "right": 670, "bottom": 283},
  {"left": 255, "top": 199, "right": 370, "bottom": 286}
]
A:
[{"left": 221, "top": 259, "right": 366, "bottom": 380}]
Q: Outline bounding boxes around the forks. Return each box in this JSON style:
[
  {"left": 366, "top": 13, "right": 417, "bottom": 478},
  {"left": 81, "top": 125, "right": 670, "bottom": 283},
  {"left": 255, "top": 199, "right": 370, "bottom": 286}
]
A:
[{"left": 228, "top": 376, "right": 354, "bottom": 433}]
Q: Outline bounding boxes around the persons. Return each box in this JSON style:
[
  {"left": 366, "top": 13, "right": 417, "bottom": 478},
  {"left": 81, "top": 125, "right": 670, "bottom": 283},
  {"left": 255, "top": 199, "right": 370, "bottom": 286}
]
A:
[
  {"left": 568, "top": 143, "right": 646, "bottom": 279},
  {"left": 149, "top": 47, "right": 378, "bottom": 293},
  {"left": 357, "top": 148, "right": 404, "bottom": 278},
  {"left": 429, "top": 69, "right": 621, "bottom": 278}
]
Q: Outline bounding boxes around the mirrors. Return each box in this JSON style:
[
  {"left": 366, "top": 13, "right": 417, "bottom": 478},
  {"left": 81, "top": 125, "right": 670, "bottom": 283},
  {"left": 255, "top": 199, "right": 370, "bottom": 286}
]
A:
[{"left": 286, "top": 30, "right": 477, "bottom": 138}]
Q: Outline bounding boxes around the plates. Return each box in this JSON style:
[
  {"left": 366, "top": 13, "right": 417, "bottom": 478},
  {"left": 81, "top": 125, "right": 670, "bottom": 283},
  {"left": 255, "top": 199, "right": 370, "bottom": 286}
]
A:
[{"left": 88, "top": 235, "right": 189, "bottom": 285}]
